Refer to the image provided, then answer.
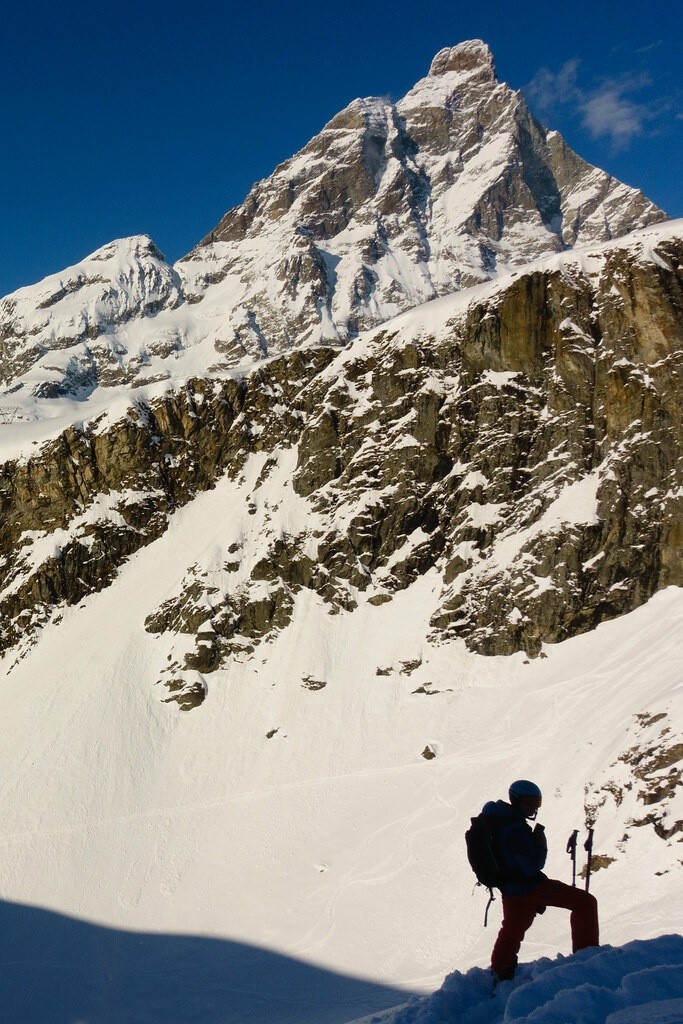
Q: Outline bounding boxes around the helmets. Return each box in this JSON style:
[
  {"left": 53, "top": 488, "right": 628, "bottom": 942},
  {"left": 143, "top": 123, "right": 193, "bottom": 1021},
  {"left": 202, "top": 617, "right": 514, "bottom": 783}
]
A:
[{"left": 508, "top": 779, "right": 543, "bottom": 812}]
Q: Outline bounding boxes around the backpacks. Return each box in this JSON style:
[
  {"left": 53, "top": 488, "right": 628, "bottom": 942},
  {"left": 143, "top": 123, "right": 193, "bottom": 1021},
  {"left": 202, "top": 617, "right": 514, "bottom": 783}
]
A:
[{"left": 465, "top": 799, "right": 508, "bottom": 888}]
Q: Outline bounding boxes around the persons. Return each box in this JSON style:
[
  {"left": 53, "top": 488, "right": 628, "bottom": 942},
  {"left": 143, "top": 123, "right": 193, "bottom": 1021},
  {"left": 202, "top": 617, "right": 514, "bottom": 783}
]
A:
[{"left": 464, "top": 779, "right": 600, "bottom": 983}]
